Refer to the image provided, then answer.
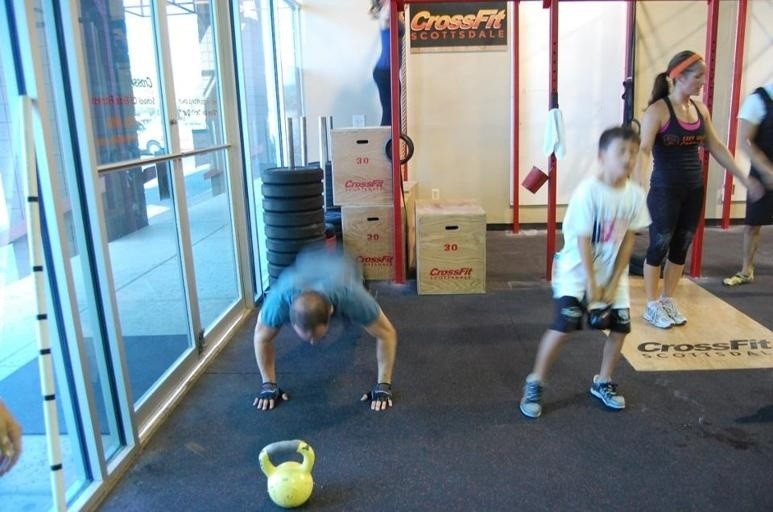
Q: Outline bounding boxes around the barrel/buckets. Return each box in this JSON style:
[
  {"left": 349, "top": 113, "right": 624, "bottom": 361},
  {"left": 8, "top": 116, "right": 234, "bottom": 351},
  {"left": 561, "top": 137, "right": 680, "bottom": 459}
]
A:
[{"left": 521, "top": 154, "right": 554, "bottom": 193}]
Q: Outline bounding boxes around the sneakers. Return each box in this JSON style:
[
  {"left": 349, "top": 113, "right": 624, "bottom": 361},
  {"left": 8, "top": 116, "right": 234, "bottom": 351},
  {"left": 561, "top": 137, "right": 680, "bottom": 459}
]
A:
[
  {"left": 590, "top": 374, "right": 625, "bottom": 409},
  {"left": 642, "top": 302, "right": 675, "bottom": 329},
  {"left": 520, "top": 380, "right": 544, "bottom": 418},
  {"left": 663, "top": 299, "right": 687, "bottom": 326},
  {"left": 723, "top": 271, "right": 754, "bottom": 286}
]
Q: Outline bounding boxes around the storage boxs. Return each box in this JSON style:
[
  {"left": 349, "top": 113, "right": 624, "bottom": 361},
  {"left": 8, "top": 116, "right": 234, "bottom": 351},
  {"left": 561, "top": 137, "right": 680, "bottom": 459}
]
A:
[
  {"left": 330, "top": 126, "right": 399, "bottom": 206},
  {"left": 340, "top": 194, "right": 410, "bottom": 280},
  {"left": 403, "top": 181, "right": 419, "bottom": 202},
  {"left": 416, "top": 198, "right": 489, "bottom": 296}
]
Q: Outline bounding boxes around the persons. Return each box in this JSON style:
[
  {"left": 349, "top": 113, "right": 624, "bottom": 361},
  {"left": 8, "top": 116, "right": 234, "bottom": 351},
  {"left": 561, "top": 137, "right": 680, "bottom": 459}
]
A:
[
  {"left": 519, "top": 126, "right": 653, "bottom": 418},
  {"left": 253, "top": 239, "right": 397, "bottom": 411},
  {"left": 0, "top": 402, "right": 22, "bottom": 475},
  {"left": 368, "top": 0, "right": 405, "bottom": 126},
  {"left": 721, "top": 83, "right": 773, "bottom": 287},
  {"left": 638, "top": 49, "right": 765, "bottom": 329}
]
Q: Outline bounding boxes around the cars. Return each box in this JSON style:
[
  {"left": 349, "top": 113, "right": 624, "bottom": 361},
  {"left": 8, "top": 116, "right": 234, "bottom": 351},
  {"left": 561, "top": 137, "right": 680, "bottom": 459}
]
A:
[{"left": 133, "top": 112, "right": 164, "bottom": 155}]
